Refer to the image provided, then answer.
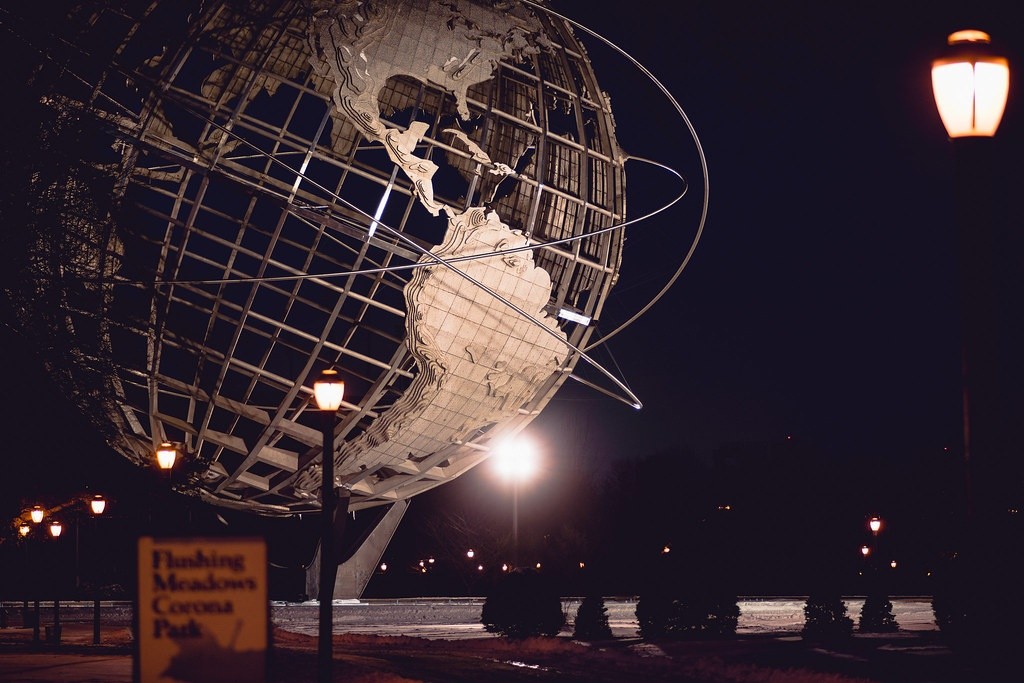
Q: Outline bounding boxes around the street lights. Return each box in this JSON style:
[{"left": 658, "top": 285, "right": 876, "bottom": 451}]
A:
[
  {"left": 18, "top": 521, "right": 29, "bottom": 631},
  {"left": 869, "top": 516, "right": 883, "bottom": 565},
  {"left": 860, "top": 544, "right": 870, "bottom": 569},
  {"left": 89, "top": 493, "right": 108, "bottom": 646},
  {"left": 485, "top": 431, "right": 551, "bottom": 568},
  {"left": 47, "top": 521, "right": 66, "bottom": 641},
  {"left": 29, "top": 504, "right": 45, "bottom": 642},
  {"left": 310, "top": 364, "right": 346, "bottom": 663},
  {"left": 923, "top": 26, "right": 1012, "bottom": 519}
]
